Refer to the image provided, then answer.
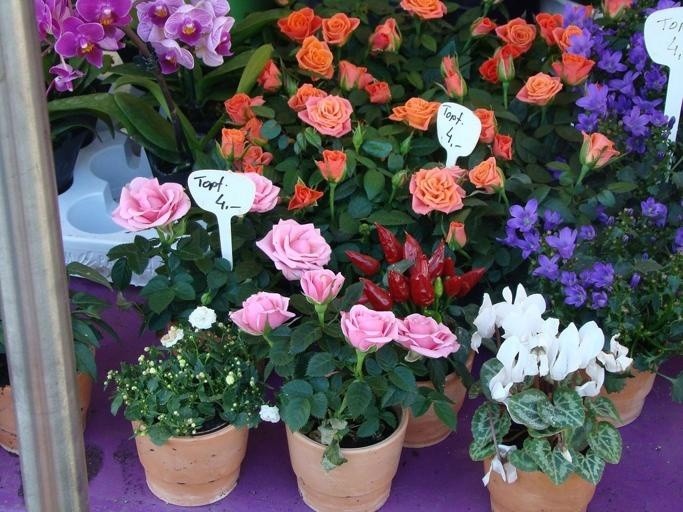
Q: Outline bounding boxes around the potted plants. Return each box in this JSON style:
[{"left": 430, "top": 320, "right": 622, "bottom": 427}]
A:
[{"left": 0, "top": 261, "right": 116, "bottom": 459}]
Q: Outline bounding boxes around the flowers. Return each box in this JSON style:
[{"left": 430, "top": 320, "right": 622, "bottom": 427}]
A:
[
  {"left": 31, "top": 3, "right": 683, "bottom": 360},
  {"left": 465, "top": 284, "right": 632, "bottom": 487},
  {"left": 103, "top": 296, "right": 280, "bottom": 441}
]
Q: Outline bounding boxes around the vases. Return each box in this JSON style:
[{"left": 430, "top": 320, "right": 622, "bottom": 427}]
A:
[
  {"left": 407, "top": 352, "right": 474, "bottom": 450},
  {"left": 131, "top": 411, "right": 251, "bottom": 507},
  {"left": 593, "top": 369, "right": 659, "bottom": 430},
  {"left": 482, "top": 450, "right": 599, "bottom": 512},
  {"left": 283, "top": 407, "right": 408, "bottom": 509}
]
